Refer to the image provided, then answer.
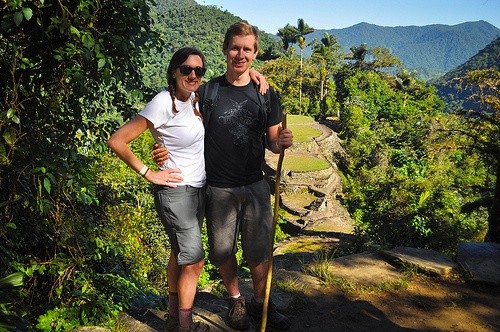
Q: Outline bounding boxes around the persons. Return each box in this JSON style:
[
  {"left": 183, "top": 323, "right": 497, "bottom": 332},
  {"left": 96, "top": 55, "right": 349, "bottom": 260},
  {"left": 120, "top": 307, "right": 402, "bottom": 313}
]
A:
[
  {"left": 150, "top": 22, "right": 294, "bottom": 332},
  {"left": 108, "top": 47, "right": 269, "bottom": 332}
]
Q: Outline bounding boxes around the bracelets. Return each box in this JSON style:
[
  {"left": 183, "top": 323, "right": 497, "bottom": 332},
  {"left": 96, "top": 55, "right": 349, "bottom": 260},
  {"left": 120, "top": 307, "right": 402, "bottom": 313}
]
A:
[
  {"left": 139, "top": 165, "right": 150, "bottom": 176},
  {"left": 276, "top": 140, "right": 280, "bottom": 151}
]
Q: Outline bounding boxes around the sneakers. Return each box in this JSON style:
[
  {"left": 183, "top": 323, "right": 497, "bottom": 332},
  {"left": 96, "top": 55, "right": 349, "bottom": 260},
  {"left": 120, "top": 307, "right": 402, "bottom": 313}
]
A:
[
  {"left": 249, "top": 296, "right": 289, "bottom": 330},
  {"left": 162, "top": 314, "right": 177, "bottom": 332},
  {"left": 227, "top": 294, "right": 250, "bottom": 331},
  {"left": 176, "top": 320, "right": 210, "bottom": 332}
]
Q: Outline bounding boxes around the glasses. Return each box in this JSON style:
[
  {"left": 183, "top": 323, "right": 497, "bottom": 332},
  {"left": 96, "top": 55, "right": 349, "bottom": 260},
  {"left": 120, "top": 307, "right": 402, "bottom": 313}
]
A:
[{"left": 177, "top": 65, "right": 207, "bottom": 77}]
